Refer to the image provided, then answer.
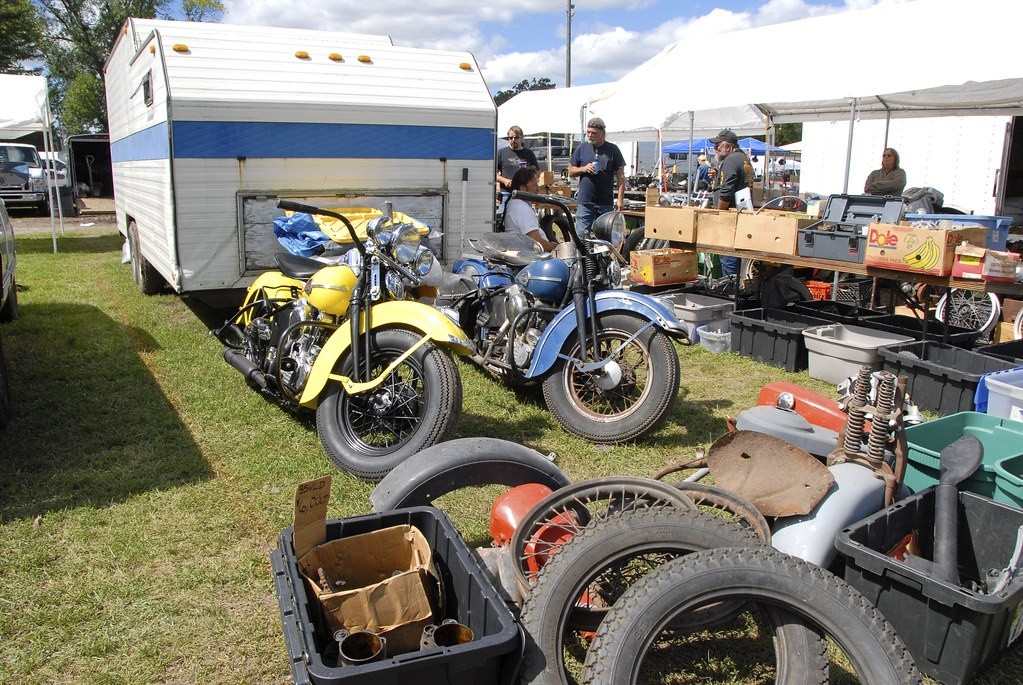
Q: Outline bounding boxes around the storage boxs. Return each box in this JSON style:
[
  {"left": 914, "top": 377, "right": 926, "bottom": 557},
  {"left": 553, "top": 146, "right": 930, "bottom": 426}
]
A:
[
  {"left": 270, "top": 506, "right": 526, "bottom": 685},
  {"left": 615, "top": 206, "right": 1023, "bottom": 685}
]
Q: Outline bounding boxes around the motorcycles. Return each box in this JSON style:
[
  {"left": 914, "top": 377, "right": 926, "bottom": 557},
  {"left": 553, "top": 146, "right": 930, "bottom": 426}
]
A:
[
  {"left": 212, "top": 200, "right": 478, "bottom": 483},
  {"left": 433, "top": 190, "right": 692, "bottom": 444}
]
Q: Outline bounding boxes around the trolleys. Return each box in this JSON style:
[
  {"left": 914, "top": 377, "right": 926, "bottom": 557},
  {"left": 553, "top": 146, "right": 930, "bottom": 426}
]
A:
[{"left": 86, "top": 155, "right": 103, "bottom": 198}]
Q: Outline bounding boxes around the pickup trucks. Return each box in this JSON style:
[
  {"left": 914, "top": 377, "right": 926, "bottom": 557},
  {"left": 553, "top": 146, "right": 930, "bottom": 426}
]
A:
[{"left": 531, "top": 145, "right": 574, "bottom": 179}]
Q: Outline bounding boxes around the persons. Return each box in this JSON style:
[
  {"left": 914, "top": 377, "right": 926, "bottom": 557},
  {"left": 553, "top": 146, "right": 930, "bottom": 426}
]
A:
[
  {"left": 504, "top": 167, "right": 558, "bottom": 255},
  {"left": 862, "top": 148, "right": 906, "bottom": 200},
  {"left": 709, "top": 130, "right": 754, "bottom": 283},
  {"left": 496, "top": 125, "right": 541, "bottom": 198},
  {"left": 569, "top": 118, "right": 627, "bottom": 241},
  {"left": 690, "top": 154, "right": 713, "bottom": 187}
]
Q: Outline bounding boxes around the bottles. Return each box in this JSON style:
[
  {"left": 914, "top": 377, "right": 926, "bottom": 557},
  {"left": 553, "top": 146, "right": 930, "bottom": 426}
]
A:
[{"left": 591, "top": 150, "right": 599, "bottom": 174}]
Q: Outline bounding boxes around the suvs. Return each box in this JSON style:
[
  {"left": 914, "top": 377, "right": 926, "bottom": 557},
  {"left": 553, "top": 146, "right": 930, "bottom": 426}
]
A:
[{"left": 0, "top": 142, "right": 48, "bottom": 217}]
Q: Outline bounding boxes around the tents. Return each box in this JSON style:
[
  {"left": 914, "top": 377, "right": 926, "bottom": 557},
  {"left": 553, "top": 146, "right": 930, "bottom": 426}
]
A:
[
  {"left": 0, "top": 72, "right": 65, "bottom": 252},
  {"left": 492, "top": 0, "right": 1023, "bottom": 301}
]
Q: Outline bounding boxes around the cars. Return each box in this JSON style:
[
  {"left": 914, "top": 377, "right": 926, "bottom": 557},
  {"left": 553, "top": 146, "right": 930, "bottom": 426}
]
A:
[
  {"left": 42, "top": 159, "right": 70, "bottom": 196},
  {"left": 0, "top": 197, "right": 17, "bottom": 320}
]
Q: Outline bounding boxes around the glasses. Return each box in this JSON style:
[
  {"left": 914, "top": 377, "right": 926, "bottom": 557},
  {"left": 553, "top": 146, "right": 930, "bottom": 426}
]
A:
[
  {"left": 508, "top": 136, "right": 520, "bottom": 140},
  {"left": 883, "top": 154, "right": 892, "bottom": 157}
]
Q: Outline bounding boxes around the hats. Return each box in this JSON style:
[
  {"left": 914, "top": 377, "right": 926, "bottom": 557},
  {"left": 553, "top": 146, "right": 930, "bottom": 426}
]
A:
[
  {"left": 587, "top": 117, "right": 606, "bottom": 130},
  {"left": 709, "top": 130, "right": 738, "bottom": 144}
]
